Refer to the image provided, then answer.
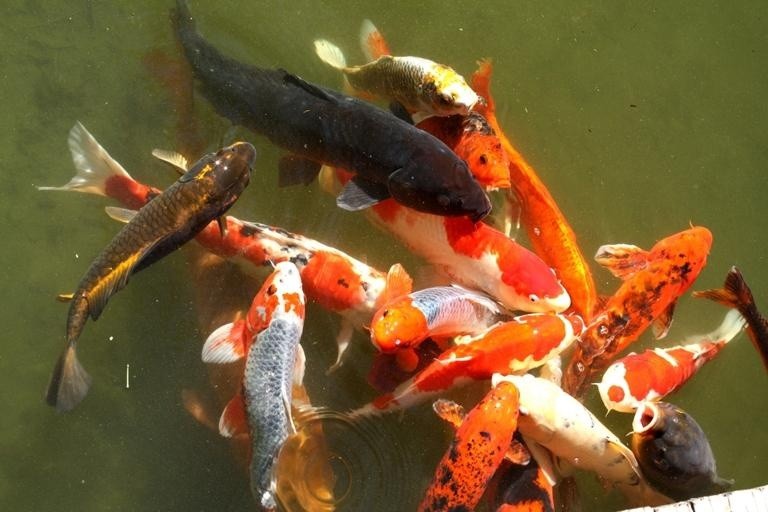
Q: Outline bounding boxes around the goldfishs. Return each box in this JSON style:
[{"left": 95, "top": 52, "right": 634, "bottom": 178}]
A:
[{"left": 141, "top": 0, "right": 768, "bottom": 512}]
